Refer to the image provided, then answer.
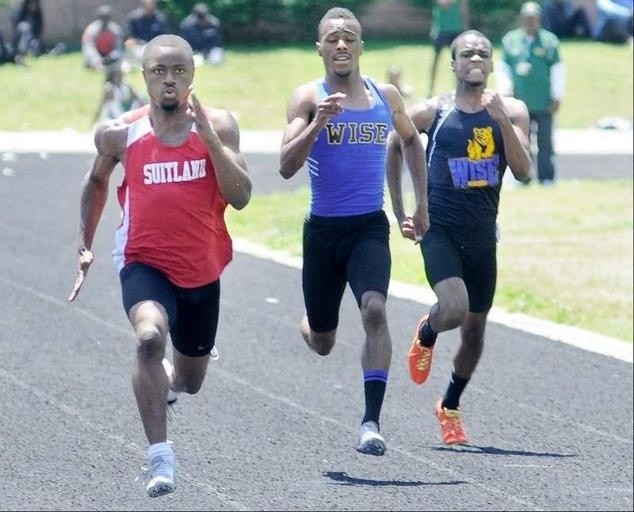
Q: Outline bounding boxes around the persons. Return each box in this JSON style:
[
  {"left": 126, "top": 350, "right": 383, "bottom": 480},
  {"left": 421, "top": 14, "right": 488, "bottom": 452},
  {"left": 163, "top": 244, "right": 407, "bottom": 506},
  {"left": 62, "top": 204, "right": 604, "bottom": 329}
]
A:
[
  {"left": 277, "top": 8, "right": 430, "bottom": 455},
  {"left": 428, "top": 0, "right": 472, "bottom": 101},
  {"left": 386, "top": 29, "right": 533, "bottom": 447},
  {"left": 388, "top": 66, "right": 415, "bottom": 110},
  {"left": 542, "top": 0, "right": 592, "bottom": 40},
  {"left": 0, "top": 0, "right": 224, "bottom": 128},
  {"left": 495, "top": 0, "right": 563, "bottom": 187},
  {"left": 67, "top": 33, "right": 253, "bottom": 497}
]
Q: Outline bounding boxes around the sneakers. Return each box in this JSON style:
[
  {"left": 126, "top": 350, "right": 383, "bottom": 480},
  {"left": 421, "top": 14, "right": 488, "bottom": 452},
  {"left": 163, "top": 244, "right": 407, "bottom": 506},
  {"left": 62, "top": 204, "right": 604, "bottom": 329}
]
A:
[
  {"left": 408, "top": 316, "right": 436, "bottom": 384},
  {"left": 147, "top": 453, "right": 177, "bottom": 497},
  {"left": 437, "top": 398, "right": 468, "bottom": 447},
  {"left": 161, "top": 360, "right": 177, "bottom": 427},
  {"left": 353, "top": 422, "right": 386, "bottom": 456}
]
